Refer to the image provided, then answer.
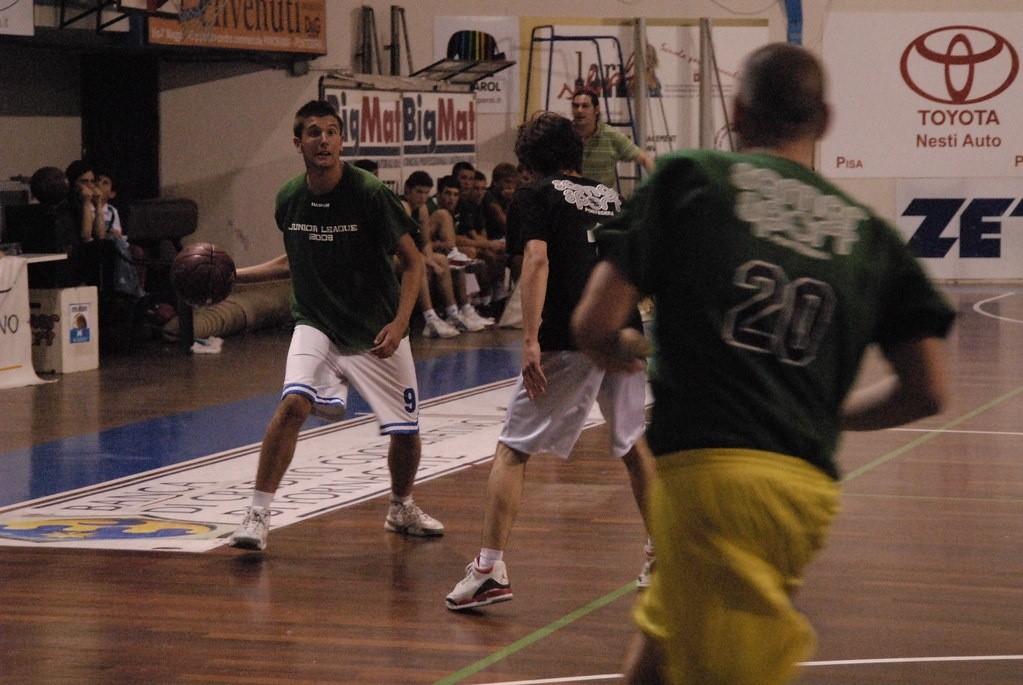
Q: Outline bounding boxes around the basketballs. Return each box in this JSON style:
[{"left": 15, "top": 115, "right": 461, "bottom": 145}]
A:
[
  {"left": 172, "top": 242, "right": 237, "bottom": 307},
  {"left": 31, "top": 165, "right": 69, "bottom": 204}
]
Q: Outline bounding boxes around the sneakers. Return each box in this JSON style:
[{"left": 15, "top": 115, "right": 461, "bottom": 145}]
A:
[
  {"left": 228, "top": 505, "right": 271, "bottom": 550},
  {"left": 459, "top": 306, "right": 495, "bottom": 325},
  {"left": 423, "top": 317, "right": 460, "bottom": 339},
  {"left": 384, "top": 497, "right": 444, "bottom": 535},
  {"left": 446, "top": 556, "right": 513, "bottom": 609},
  {"left": 445, "top": 310, "right": 484, "bottom": 332},
  {"left": 636, "top": 541, "right": 657, "bottom": 588}
]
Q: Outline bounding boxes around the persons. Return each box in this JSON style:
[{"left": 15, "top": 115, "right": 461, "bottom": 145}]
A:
[
  {"left": 446, "top": 111, "right": 658, "bottom": 607},
  {"left": 230, "top": 532, "right": 443, "bottom": 685},
  {"left": 568, "top": 88, "right": 657, "bottom": 198},
  {"left": 352, "top": 158, "right": 518, "bottom": 337},
  {"left": 57, "top": 157, "right": 150, "bottom": 302},
  {"left": 570, "top": 41, "right": 960, "bottom": 685},
  {"left": 229, "top": 98, "right": 445, "bottom": 550}
]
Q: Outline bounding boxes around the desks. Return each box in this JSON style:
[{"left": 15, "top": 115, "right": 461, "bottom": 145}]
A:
[{"left": 11, "top": 252, "right": 68, "bottom": 264}]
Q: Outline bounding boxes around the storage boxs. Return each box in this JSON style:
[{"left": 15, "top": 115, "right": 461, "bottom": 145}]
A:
[{"left": 29, "top": 286, "right": 99, "bottom": 374}]
